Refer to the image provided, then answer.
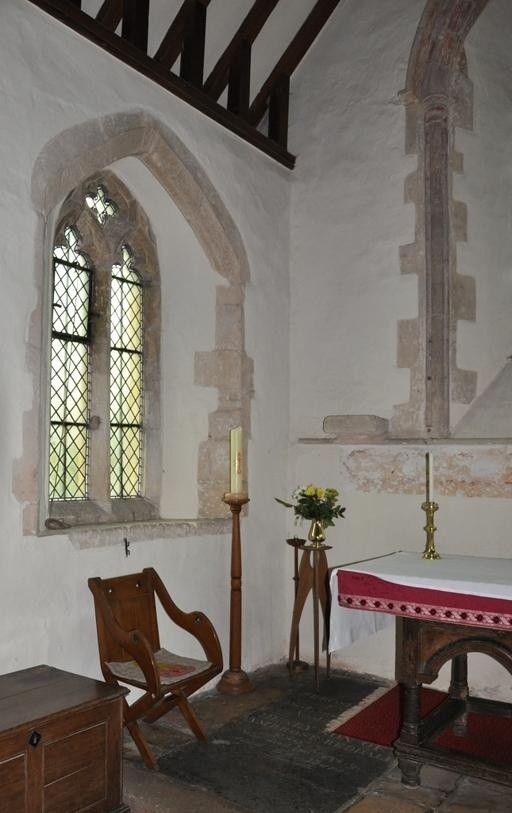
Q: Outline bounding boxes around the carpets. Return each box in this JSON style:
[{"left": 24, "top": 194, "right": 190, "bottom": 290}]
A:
[
  {"left": 323, "top": 678, "right": 510, "bottom": 768},
  {"left": 155, "top": 673, "right": 398, "bottom": 812}
]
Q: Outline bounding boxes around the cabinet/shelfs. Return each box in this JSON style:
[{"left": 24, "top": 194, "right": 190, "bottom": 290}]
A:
[{"left": 0, "top": 664, "right": 130, "bottom": 813}]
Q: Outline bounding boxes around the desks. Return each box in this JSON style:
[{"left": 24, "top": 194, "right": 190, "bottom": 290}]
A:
[{"left": 332, "top": 550, "right": 512, "bottom": 789}]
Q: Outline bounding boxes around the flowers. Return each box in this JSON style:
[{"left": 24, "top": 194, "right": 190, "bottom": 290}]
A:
[{"left": 275, "top": 483, "right": 346, "bottom": 530}]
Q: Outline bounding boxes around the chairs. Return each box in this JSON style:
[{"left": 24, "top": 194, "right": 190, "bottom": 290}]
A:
[{"left": 88, "top": 567, "right": 223, "bottom": 770}]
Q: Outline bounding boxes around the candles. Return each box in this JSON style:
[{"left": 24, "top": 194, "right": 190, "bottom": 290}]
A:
[
  {"left": 230, "top": 426, "right": 243, "bottom": 494},
  {"left": 428, "top": 451, "right": 433, "bottom": 502}
]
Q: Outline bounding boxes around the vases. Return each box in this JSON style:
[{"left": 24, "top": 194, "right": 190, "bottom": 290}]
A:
[{"left": 309, "top": 518, "right": 325, "bottom": 548}]
